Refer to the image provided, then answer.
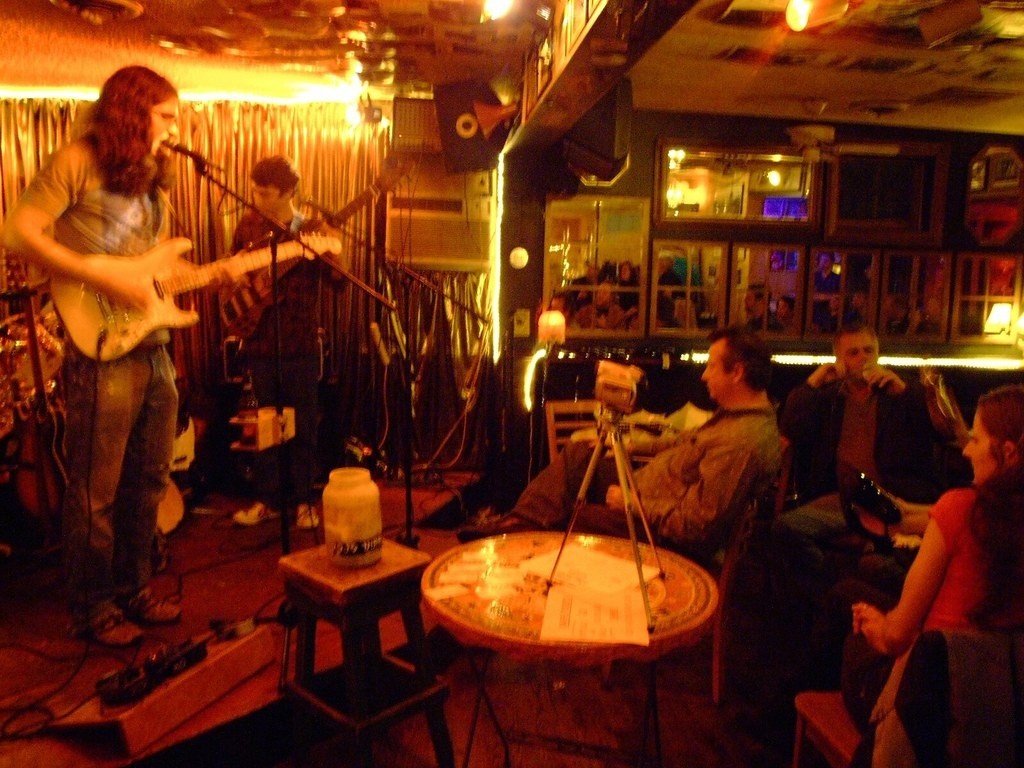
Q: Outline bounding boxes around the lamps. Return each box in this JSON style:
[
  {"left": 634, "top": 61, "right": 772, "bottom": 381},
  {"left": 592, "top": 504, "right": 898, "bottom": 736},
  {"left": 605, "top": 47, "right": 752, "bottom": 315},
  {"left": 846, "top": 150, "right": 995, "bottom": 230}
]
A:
[{"left": 785, "top": 0, "right": 849, "bottom": 32}]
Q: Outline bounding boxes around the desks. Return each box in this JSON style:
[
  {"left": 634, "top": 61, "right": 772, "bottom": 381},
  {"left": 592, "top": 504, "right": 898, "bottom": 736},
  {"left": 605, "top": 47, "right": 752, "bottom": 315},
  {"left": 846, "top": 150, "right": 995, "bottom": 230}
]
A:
[{"left": 420, "top": 532, "right": 722, "bottom": 768}]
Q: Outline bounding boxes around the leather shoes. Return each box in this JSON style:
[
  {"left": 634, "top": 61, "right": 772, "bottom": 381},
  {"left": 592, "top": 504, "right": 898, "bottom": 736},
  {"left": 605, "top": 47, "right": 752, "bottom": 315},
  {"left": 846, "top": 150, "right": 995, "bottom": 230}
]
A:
[{"left": 456, "top": 511, "right": 541, "bottom": 543}]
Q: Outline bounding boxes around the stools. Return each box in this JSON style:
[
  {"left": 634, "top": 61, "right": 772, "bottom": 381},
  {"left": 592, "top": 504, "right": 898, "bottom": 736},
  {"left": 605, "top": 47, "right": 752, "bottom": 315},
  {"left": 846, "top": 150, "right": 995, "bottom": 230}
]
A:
[{"left": 275, "top": 537, "right": 458, "bottom": 768}]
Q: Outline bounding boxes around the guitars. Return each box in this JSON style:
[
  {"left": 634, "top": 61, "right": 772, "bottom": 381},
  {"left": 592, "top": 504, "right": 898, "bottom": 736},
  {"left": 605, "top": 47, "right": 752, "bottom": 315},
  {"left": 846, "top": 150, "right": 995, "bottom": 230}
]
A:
[
  {"left": 218, "top": 151, "right": 416, "bottom": 339},
  {"left": 14, "top": 264, "right": 68, "bottom": 519},
  {"left": 49, "top": 231, "right": 342, "bottom": 364}
]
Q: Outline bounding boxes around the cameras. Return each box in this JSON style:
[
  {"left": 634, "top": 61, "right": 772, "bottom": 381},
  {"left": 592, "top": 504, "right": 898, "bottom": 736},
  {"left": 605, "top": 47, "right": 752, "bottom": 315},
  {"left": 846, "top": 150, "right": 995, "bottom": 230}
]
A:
[{"left": 595, "top": 360, "right": 646, "bottom": 414}]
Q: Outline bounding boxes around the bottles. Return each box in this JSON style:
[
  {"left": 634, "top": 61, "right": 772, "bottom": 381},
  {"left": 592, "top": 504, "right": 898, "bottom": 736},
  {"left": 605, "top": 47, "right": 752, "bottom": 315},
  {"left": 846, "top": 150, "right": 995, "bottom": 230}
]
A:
[{"left": 324, "top": 467, "right": 383, "bottom": 568}]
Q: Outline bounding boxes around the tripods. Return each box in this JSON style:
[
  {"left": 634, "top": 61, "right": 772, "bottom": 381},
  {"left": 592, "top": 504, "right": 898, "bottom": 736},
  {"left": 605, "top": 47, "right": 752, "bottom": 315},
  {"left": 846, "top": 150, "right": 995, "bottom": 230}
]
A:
[{"left": 541, "top": 407, "right": 665, "bottom": 632}]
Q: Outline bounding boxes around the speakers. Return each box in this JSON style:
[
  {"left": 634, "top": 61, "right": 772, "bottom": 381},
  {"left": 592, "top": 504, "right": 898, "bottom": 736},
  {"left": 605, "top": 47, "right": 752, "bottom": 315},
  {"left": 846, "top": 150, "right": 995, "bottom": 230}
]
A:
[
  {"left": 431, "top": 76, "right": 510, "bottom": 175},
  {"left": 561, "top": 76, "right": 633, "bottom": 181}
]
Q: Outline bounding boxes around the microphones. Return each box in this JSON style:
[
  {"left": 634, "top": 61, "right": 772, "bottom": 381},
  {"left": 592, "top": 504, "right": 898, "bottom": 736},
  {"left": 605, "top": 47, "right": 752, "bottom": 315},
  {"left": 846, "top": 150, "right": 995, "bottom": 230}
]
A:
[
  {"left": 160, "top": 140, "right": 222, "bottom": 170},
  {"left": 292, "top": 195, "right": 305, "bottom": 234}
]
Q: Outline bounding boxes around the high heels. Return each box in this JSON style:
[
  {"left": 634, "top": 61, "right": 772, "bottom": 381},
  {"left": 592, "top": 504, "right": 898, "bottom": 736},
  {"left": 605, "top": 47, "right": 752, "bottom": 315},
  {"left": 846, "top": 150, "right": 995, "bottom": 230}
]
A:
[{"left": 840, "top": 467, "right": 904, "bottom": 559}]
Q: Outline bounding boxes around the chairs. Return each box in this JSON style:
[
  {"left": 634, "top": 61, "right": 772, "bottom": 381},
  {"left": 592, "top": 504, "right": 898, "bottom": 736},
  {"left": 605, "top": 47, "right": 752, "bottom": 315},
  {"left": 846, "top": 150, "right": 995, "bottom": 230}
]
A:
[
  {"left": 600, "top": 433, "right": 790, "bottom": 704},
  {"left": 545, "top": 400, "right": 600, "bottom": 463},
  {"left": 791, "top": 629, "right": 1024, "bottom": 768}
]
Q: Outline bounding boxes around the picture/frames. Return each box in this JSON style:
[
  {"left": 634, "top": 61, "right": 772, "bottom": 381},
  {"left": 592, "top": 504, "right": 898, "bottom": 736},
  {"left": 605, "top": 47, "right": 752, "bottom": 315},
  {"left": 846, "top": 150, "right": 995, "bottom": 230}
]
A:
[{"left": 653, "top": 135, "right": 824, "bottom": 235}]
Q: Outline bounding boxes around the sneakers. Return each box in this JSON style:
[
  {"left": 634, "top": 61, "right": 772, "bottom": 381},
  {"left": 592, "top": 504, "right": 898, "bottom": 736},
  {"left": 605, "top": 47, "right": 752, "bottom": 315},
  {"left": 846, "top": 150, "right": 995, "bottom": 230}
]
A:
[
  {"left": 296, "top": 503, "right": 318, "bottom": 528},
  {"left": 109, "top": 585, "right": 182, "bottom": 627},
  {"left": 232, "top": 502, "right": 282, "bottom": 526},
  {"left": 61, "top": 597, "right": 145, "bottom": 647}
]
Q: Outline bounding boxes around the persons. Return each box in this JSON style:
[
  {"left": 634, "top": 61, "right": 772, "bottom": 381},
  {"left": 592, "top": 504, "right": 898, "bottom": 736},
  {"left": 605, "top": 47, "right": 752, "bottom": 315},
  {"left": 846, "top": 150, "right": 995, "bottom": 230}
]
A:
[
  {"left": 0, "top": 65, "right": 250, "bottom": 649},
  {"left": 227, "top": 155, "right": 349, "bottom": 528},
  {"left": 547, "top": 255, "right": 919, "bottom": 335},
  {"left": 769, "top": 320, "right": 941, "bottom": 653},
  {"left": 455, "top": 325, "right": 782, "bottom": 565},
  {"left": 841, "top": 385, "right": 1024, "bottom": 768}
]
